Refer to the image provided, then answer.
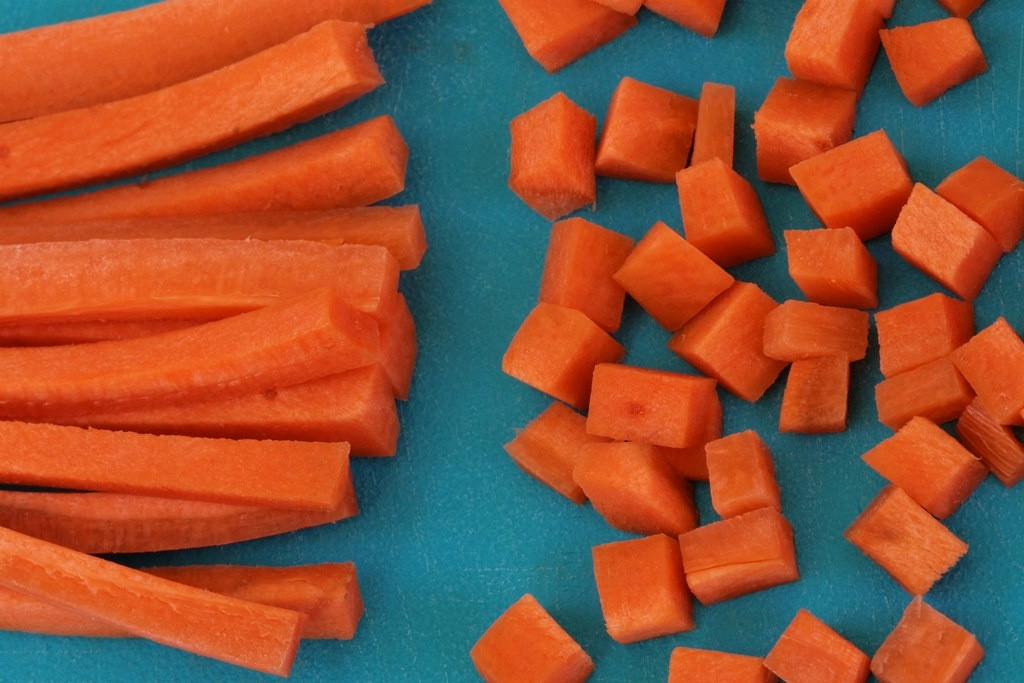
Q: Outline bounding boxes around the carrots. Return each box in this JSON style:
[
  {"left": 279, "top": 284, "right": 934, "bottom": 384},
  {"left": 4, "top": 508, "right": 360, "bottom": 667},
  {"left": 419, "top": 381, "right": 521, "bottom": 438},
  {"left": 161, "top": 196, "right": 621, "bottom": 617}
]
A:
[{"left": 0, "top": 0, "right": 1024, "bottom": 682}]
